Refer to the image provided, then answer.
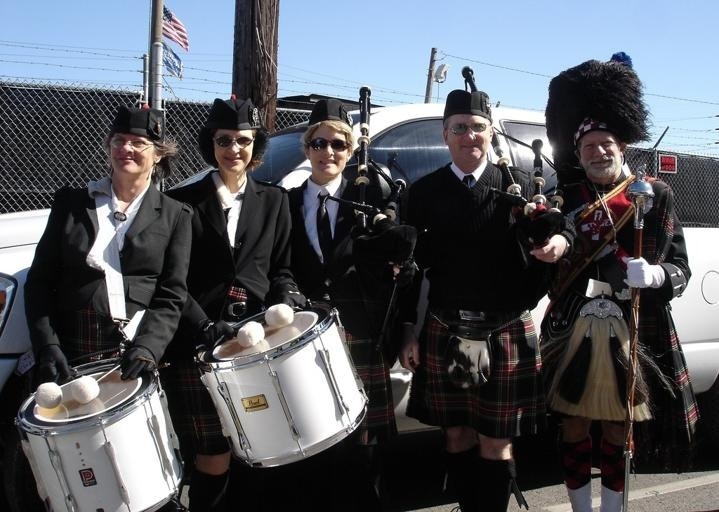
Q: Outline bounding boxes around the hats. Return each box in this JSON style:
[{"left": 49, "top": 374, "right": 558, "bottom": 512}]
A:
[
  {"left": 572, "top": 117, "right": 609, "bottom": 147},
  {"left": 206, "top": 95, "right": 263, "bottom": 131},
  {"left": 310, "top": 100, "right": 354, "bottom": 127},
  {"left": 112, "top": 106, "right": 166, "bottom": 143}
]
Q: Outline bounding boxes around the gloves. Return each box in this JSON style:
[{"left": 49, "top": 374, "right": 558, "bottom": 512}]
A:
[
  {"left": 118, "top": 346, "right": 156, "bottom": 380},
  {"left": 39, "top": 345, "right": 71, "bottom": 380},
  {"left": 622, "top": 256, "right": 666, "bottom": 289},
  {"left": 276, "top": 278, "right": 306, "bottom": 313},
  {"left": 194, "top": 320, "right": 236, "bottom": 347}
]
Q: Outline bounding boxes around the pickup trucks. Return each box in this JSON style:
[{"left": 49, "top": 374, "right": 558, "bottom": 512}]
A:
[{"left": 0, "top": 101, "right": 717, "bottom": 428}]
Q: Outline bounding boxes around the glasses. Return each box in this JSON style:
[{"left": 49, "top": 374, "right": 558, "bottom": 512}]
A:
[
  {"left": 110, "top": 137, "right": 155, "bottom": 149},
  {"left": 212, "top": 134, "right": 256, "bottom": 147},
  {"left": 442, "top": 90, "right": 492, "bottom": 124},
  {"left": 448, "top": 123, "right": 487, "bottom": 134},
  {"left": 307, "top": 139, "right": 351, "bottom": 152}
]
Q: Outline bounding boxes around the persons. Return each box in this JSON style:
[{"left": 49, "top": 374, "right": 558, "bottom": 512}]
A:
[
  {"left": 165, "top": 95, "right": 310, "bottom": 511},
  {"left": 289, "top": 100, "right": 416, "bottom": 510},
  {"left": 397, "top": 89, "right": 582, "bottom": 511},
  {"left": 0, "top": 105, "right": 194, "bottom": 508},
  {"left": 542, "top": 115, "right": 701, "bottom": 512}
]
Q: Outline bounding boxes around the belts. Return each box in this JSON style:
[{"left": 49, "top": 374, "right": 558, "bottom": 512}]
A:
[
  {"left": 457, "top": 311, "right": 485, "bottom": 323},
  {"left": 228, "top": 301, "right": 248, "bottom": 318}
]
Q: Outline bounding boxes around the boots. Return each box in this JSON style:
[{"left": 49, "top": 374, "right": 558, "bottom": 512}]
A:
[
  {"left": 444, "top": 446, "right": 482, "bottom": 512},
  {"left": 480, "top": 457, "right": 516, "bottom": 512},
  {"left": 599, "top": 482, "right": 623, "bottom": 512},
  {"left": 188, "top": 466, "right": 229, "bottom": 512},
  {"left": 562, "top": 479, "right": 592, "bottom": 512}
]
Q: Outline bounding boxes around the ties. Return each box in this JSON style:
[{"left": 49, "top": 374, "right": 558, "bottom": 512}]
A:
[
  {"left": 318, "top": 193, "right": 335, "bottom": 265},
  {"left": 463, "top": 174, "right": 473, "bottom": 186}
]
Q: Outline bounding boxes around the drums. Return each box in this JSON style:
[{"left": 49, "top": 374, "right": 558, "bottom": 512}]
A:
[
  {"left": 194, "top": 300, "right": 369, "bottom": 469},
  {"left": 16, "top": 357, "right": 187, "bottom": 512}
]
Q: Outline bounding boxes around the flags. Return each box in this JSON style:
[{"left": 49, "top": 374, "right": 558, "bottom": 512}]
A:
[{"left": 158, "top": 10, "right": 189, "bottom": 80}]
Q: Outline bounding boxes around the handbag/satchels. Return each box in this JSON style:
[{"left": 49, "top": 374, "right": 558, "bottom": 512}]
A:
[{"left": 443, "top": 323, "right": 494, "bottom": 390}]
